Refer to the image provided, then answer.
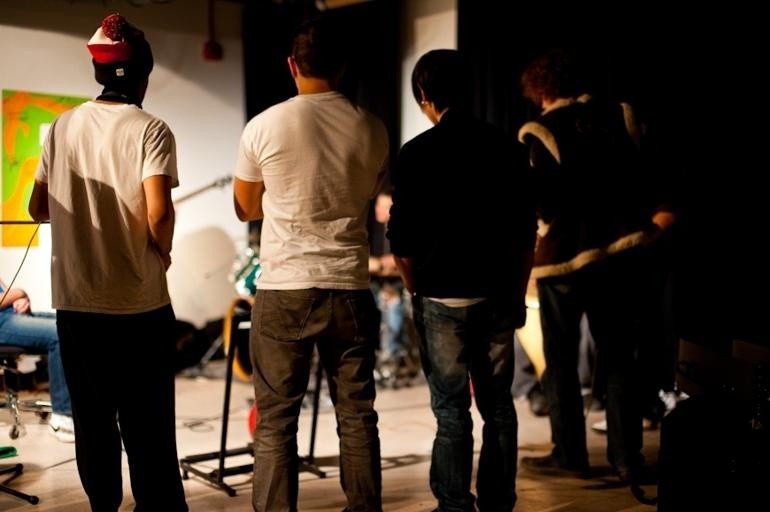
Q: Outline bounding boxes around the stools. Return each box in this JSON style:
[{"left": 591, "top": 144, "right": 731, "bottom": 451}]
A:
[{"left": 0, "top": 345, "right": 52, "bottom": 439}]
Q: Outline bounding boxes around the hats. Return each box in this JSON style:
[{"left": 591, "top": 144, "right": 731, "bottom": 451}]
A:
[{"left": 87, "top": 13, "right": 153, "bottom": 67}]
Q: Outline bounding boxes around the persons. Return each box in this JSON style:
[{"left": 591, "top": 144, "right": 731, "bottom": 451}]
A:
[
  {"left": 234, "top": 28, "right": 391, "bottom": 512},
  {"left": 514, "top": 53, "right": 661, "bottom": 482},
  {"left": 387, "top": 49, "right": 539, "bottom": 512},
  {"left": 29, "top": 14, "right": 189, "bottom": 512},
  {"left": 0, "top": 275, "right": 77, "bottom": 435}
]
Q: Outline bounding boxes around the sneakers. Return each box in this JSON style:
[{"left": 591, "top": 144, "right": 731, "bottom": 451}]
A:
[
  {"left": 518, "top": 447, "right": 593, "bottom": 479},
  {"left": 618, "top": 454, "right": 646, "bottom": 482}
]
